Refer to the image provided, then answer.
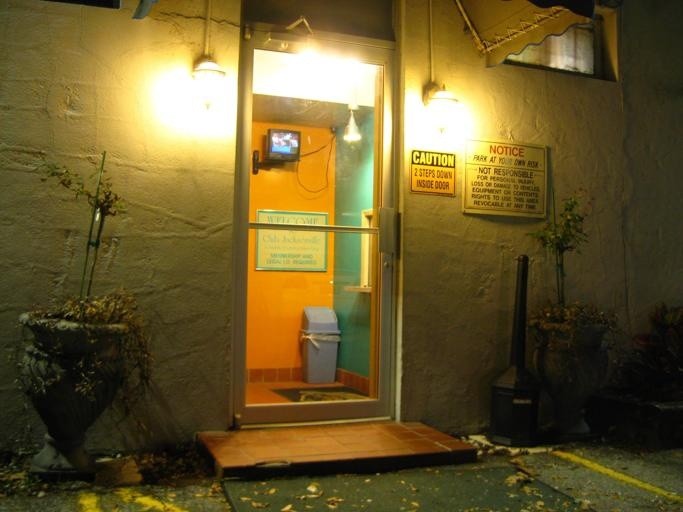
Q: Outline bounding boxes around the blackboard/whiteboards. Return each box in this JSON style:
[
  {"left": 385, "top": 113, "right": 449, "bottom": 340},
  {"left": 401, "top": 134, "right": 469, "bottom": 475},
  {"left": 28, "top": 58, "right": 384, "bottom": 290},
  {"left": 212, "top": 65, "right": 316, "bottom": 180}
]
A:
[{"left": 255, "top": 209, "right": 326, "bottom": 272}]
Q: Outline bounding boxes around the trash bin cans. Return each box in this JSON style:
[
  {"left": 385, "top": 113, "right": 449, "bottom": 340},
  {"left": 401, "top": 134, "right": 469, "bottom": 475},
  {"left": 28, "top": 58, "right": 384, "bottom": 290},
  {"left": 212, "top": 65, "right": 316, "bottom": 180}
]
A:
[{"left": 300, "top": 306, "right": 342, "bottom": 384}]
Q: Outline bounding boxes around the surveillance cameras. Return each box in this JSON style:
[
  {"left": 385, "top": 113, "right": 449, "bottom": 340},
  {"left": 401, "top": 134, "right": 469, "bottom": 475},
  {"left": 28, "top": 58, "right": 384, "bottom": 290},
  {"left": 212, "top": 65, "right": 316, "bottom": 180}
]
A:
[{"left": 330, "top": 127, "right": 336, "bottom": 133}]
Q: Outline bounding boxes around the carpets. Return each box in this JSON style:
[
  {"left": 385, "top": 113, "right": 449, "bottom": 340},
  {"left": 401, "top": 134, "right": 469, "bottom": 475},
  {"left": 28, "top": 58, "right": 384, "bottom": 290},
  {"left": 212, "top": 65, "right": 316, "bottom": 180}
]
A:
[
  {"left": 270, "top": 385, "right": 369, "bottom": 404},
  {"left": 221, "top": 464, "right": 580, "bottom": 511}
]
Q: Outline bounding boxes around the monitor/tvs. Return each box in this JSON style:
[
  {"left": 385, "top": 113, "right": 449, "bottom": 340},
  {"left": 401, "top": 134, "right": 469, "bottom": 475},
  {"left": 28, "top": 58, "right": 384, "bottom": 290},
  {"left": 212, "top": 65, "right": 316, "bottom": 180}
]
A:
[{"left": 264, "top": 128, "right": 301, "bottom": 162}]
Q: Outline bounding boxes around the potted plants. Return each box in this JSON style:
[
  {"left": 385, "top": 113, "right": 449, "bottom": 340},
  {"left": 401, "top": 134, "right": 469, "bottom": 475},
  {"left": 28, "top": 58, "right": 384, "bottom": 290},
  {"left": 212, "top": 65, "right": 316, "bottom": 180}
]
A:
[
  {"left": 612, "top": 302, "right": 681, "bottom": 454},
  {"left": 17, "top": 147, "right": 157, "bottom": 478},
  {"left": 528, "top": 195, "right": 617, "bottom": 427}
]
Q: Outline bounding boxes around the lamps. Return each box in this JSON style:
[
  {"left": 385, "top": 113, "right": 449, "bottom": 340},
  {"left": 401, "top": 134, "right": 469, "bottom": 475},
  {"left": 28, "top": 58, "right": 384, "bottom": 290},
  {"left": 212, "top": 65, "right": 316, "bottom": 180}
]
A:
[{"left": 339, "top": 106, "right": 362, "bottom": 143}]
